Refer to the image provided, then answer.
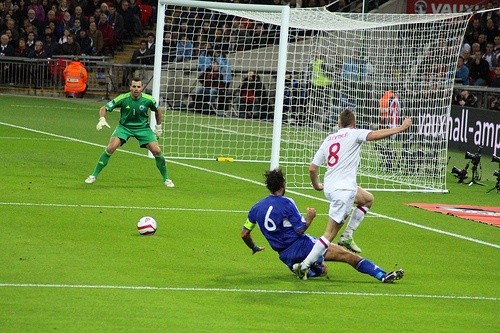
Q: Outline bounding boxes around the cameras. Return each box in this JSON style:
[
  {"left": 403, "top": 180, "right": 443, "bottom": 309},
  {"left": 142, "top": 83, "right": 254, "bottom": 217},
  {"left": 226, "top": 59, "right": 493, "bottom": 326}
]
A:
[
  {"left": 451, "top": 163, "right": 469, "bottom": 183},
  {"left": 491, "top": 154, "right": 500, "bottom": 179},
  {"left": 465, "top": 146, "right": 483, "bottom": 171}
]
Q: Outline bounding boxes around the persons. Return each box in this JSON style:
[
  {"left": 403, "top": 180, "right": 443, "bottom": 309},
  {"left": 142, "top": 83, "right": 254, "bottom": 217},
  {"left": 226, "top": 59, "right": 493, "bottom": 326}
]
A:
[
  {"left": 85, "top": 79, "right": 174, "bottom": 188},
  {"left": 292, "top": 109, "right": 412, "bottom": 280},
  {"left": 130, "top": 0, "right": 500, "bottom": 140},
  {"left": 63, "top": 56, "right": 88, "bottom": 98},
  {"left": 379, "top": 82, "right": 402, "bottom": 140},
  {"left": 0, "top": 0, "right": 145, "bottom": 90},
  {"left": 240, "top": 168, "right": 404, "bottom": 283}
]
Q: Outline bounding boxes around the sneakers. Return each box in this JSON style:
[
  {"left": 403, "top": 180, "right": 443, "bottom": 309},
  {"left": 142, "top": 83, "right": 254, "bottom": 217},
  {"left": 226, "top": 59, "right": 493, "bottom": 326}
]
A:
[
  {"left": 85, "top": 175, "right": 96, "bottom": 183},
  {"left": 164, "top": 179, "right": 175, "bottom": 187},
  {"left": 292, "top": 264, "right": 308, "bottom": 281},
  {"left": 382, "top": 269, "right": 404, "bottom": 282},
  {"left": 338, "top": 235, "right": 362, "bottom": 253}
]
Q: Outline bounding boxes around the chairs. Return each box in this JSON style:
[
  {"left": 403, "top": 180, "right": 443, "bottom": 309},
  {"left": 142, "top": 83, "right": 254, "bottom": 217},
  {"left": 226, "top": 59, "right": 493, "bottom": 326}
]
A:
[{"left": 103, "top": 15, "right": 141, "bottom": 58}]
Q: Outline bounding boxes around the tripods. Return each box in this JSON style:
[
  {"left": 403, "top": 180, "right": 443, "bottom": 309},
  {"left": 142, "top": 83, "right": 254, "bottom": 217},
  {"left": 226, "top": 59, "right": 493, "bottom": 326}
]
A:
[
  {"left": 467, "top": 165, "right": 486, "bottom": 186},
  {"left": 486, "top": 178, "right": 500, "bottom": 193}
]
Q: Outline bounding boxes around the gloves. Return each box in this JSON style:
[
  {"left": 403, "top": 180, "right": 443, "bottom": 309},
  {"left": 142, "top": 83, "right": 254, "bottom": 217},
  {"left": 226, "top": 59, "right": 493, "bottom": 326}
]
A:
[
  {"left": 96, "top": 116, "right": 111, "bottom": 131},
  {"left": 154, "top": 124, "right": 162, "bottom": 136}
]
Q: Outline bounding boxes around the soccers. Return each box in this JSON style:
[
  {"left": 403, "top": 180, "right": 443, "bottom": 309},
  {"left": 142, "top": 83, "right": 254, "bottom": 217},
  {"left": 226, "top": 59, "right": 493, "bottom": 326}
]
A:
[{"left": 137, "top": 216, "right": 157, "bottom": 234}]
